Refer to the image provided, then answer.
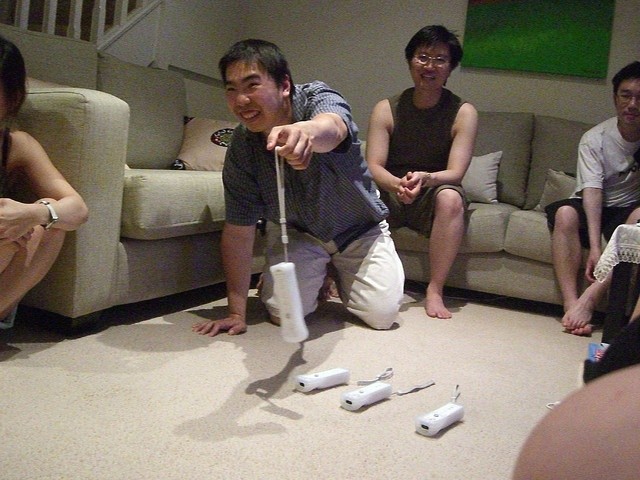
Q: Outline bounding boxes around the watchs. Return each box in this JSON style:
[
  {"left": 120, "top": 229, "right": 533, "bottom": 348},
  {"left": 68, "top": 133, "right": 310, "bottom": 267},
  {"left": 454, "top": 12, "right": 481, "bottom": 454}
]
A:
[{"left": 38, "top": 198, "right": 59, "bottom": 231}]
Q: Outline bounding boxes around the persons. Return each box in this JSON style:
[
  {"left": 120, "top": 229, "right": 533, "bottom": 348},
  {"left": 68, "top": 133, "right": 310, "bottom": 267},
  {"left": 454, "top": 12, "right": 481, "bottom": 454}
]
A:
[
  {"left": 2, "top": 33, "right": 88, "bottom": 342},
  {"left": 365, "top": 23, "right": 480, "bottom": 323},
  {"left": 509, "top": 282, "right": 639, "bottom": 480},
  {"left": 191, "top": 40, "right": 406, "bottom": 339},
  {"left": 548, "top": 61, "right": 640, "bottom": 335}
]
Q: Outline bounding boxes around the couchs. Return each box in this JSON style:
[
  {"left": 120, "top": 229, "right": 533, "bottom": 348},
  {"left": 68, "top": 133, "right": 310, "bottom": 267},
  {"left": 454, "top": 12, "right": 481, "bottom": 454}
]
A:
[
  {"left": 0, "top": 22, "right": 268, "bottom": 332},
  {"left": 391, "top": 110, "right": 610, "bottom": 316}
]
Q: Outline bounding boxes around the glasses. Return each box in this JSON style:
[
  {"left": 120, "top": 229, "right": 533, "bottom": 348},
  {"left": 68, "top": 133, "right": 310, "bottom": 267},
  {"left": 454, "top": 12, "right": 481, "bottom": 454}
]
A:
[
  {"left": 412, "top": 53, "right": 452, "bottom": 70},
  {"left": 613, "top": 91, "right": 640, "bottom": 104}
]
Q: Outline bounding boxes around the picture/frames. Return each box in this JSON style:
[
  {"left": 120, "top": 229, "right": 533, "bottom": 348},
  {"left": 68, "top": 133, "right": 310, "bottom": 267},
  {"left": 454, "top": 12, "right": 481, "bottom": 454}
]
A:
[{"left": 461, "top": 0, "right": 615, "bottom": 79}]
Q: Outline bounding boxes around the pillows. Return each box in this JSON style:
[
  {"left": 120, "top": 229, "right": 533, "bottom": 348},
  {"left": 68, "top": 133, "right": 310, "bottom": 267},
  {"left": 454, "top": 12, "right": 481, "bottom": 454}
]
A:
[
  {"left": 461, "top": 150, "right": 504, "bottom": 204},
  {"left": 98, "top": 49, "right": 186, "bottom": 170},
  {"left": 534, "top": 168, "right": 577, "bottom": 213},
  {"left": 169, "top": 115, "right": 240, "bottom": 171}
]
